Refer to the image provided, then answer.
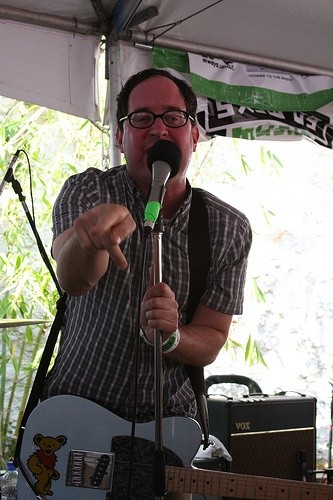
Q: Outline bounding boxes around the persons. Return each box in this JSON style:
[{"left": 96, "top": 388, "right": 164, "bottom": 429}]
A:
[{"left": 39, "top": 69, "right": 253, "bottom": 423}]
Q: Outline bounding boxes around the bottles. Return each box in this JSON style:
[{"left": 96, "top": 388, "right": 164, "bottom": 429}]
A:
[{"left": 0, "top": 462, "right": 19, "bottom": 500}]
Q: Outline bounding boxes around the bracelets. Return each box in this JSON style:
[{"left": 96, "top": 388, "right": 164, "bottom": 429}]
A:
[{"left": 140, "top": 327, "right": 180, "bottom": 354}]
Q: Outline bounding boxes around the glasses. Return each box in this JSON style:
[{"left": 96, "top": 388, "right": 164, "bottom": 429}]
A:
[{"left": 120, "top": 109, "right": 194, "bottom": 128}]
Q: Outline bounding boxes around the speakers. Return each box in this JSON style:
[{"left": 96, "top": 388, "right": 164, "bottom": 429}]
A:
[{"left": 194, "top": 394, "right": 316, "bottom": 483}]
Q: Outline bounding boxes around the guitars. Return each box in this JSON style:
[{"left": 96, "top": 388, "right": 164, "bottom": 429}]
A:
[{"left": 17, "top": 394, "right": 333, "bottom": 500}]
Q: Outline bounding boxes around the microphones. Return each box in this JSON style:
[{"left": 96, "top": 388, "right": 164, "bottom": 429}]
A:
[
  {"left": 143, "top": 140, "right": 183, "bottom": 235},
  {"left": 0, "top": 150, "right": 21, "bottom": 195}
]
